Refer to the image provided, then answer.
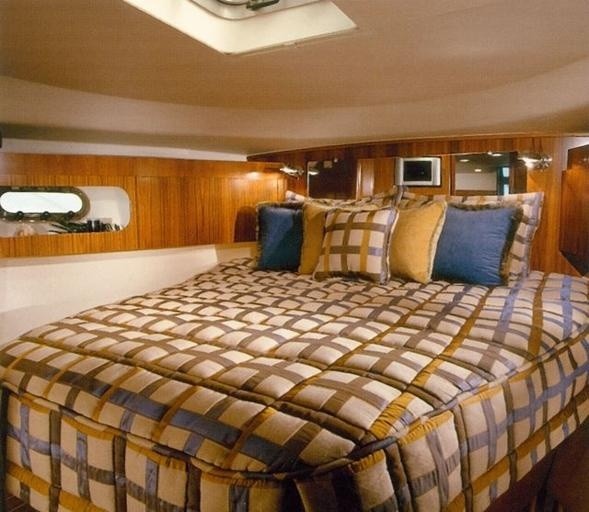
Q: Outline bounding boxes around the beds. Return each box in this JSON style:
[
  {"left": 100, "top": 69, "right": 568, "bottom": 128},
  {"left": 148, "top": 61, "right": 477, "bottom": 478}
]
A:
[{"left": 2, "top": 255, "right": 588, "bottom": 509}]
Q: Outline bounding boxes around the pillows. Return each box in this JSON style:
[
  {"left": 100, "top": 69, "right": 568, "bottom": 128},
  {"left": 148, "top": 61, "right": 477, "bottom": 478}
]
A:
[{"left": 254, "top": 189, "right": 542, "bottom": 286}]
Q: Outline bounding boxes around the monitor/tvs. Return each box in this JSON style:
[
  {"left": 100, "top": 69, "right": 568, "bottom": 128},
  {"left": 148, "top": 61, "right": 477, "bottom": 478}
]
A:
[{"left": 395, "top": 156, "right": 441, "bottom": 187}]
